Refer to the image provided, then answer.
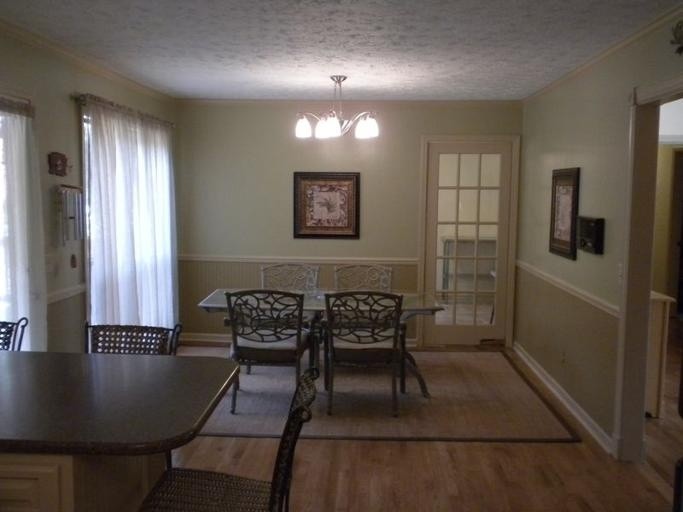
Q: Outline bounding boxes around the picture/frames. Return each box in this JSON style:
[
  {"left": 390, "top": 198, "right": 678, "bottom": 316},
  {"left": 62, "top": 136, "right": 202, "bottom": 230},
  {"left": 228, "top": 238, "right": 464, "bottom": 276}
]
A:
[
  {"left": 292, "top": 171, "right": 362, "bottom": 239},
  {"left": 547, "top": 167, "right": 580, "bottom": 261}
]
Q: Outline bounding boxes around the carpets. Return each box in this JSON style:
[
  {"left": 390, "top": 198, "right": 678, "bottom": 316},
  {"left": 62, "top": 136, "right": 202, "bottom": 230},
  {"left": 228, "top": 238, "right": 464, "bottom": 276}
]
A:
[{"left": 173, "top": 344, "right": 581, "bottom": 443}]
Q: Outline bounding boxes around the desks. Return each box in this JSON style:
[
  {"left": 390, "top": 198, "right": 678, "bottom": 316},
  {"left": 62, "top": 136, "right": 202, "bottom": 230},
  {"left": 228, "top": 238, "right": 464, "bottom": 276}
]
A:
[{"left": 194, "top": 284, "right": 446, "bottom": 399}]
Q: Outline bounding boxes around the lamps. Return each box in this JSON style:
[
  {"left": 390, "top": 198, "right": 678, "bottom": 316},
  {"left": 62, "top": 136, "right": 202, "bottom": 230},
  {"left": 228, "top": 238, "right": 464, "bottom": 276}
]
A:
[{"left": 295, "top": 75, "right": 380, "bottom": 143}]
